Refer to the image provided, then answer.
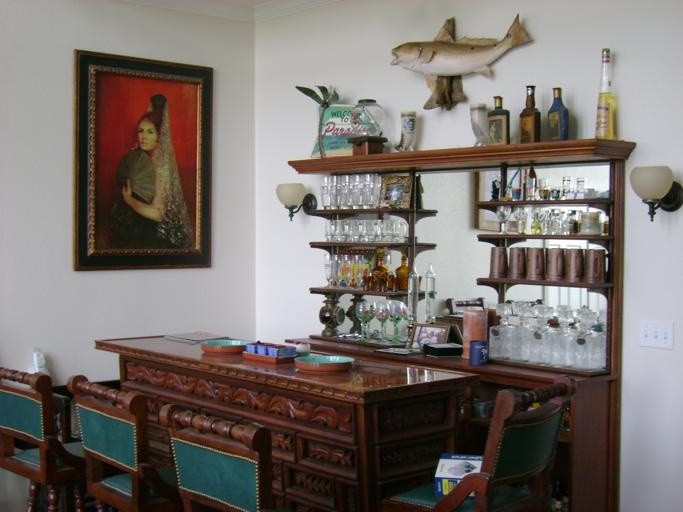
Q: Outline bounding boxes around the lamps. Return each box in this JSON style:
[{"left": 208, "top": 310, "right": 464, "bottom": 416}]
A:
[
  {"left": 275, "top": 182, "right": 317, "bottom": 221},
  {"left": 629, "top": 166, "right": 683, "bottom": 221}
]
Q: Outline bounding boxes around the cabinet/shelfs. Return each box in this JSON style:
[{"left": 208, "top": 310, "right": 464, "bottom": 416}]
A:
[{"left": 285, "top": 139, "right": 638, "bottom": 510}]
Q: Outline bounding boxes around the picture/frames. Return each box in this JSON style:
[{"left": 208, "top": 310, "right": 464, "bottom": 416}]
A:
[{"left": 72, "top": 48, "right": 214, "bottom": 271}]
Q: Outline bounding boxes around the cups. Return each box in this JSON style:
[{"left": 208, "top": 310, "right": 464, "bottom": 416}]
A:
[
  {"left": 319, "top": 173, "right": 382, "bottom": 209},
  {"left": 355, "top": 301, "right": 376, "bottom": 342},
  {"left": 549, "top": 186, "right": 561, "bottom": 200},
  {"left": 538, "top": 178, "right": 549, "bottom": 200},
  {"left": 562, "top": 176, "right": 571, "bottom": 199},
  {"left": 323, "top": 254, "right": 338, "bottom": 289},
  {"left": 524, "top": 247, "right": 545, "bottom": 280},
  {"left": 497, "top": 186, "right": 510, "bottom": 202},
  {"left": 324, "top": 220, "right": 406, "bottom": 243},
  {"left": 199, "top": 338, "right": 251, "bottom": 355},
  {"left": 388, "top": 301, "right": 406, "bottom": 345},
  {"left": 511, "top": 188, "right": 521, "bottom": 201},
  {"left": 507, "top": 246, "right": 526, "bottom": 280},
  {"left": 564, "top": 248, "right": 584, "bottom": 282},
  {"left": 353, "top": 254, "right": 364, "bottom": 290},
  {"left": 526, "top": 178, "right": 535, "bottom": 201},
  {"left": 513, "top": 204, "right": 529, "bottom": 235},
  {"left": 469, "top": 339, "right": 490, "bottom": 367},
  {"left": 488, "top": 300, "right": 607, "bottom": 370},
  {"left": 545, "top": 247, "right": 565, "bottom": 281},
  {"left": 489, "top": 246, "right": 508, "bottom": 279},
  {"left": 575, "top": 175, "right": 586, "bottom": 200},
  {"left": 338, "top": 254, "right": 353, "bottom": 289},
  {"left": 584, "top": 248, "right": 607, "bottom": 283},
  {"left": 496, "top": 204, "right": 511, "bottom": 234},
  {"left": 579, "top": 212, "right": 603, "bottom": 233},
  {"left": 374, "top": 301, "right": 391, "bottom": 344}
]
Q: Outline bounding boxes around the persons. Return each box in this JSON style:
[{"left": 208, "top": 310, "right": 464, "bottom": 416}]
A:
[{"left": 106, "top": 93, "right": 195, "bottom": 249}]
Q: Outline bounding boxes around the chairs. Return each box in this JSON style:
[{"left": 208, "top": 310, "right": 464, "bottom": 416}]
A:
[
  {"left": 0, "top": 365, "right": 120, "bottom": 511},
  {"left": 158, "top": 404, "right": 273, "bottom": 511},
  {"left": 64, "top": 374, "right": 183, "bottom": 512},
  {"left": 382, "top": 374, "right": 577, "bottom": 510}
]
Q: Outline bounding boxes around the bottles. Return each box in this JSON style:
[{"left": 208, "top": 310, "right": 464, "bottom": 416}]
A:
[
  {"left": 549, "top": 88, "right": 568, "bottom": 140},
  {"left": 596, "top": 49, "right": 619, "bottom": 141},
  {"left": 488, "top": 96, "right": 510, "bottom": 144},
  {"left": 372, "top": 253, "right": 387, "bottom": 291},
  {"left": 396, "top": 257, "right": 409, "bottom": 290},
  {"left": 520, "top": 86, "right": 541, "bottom": 143}
]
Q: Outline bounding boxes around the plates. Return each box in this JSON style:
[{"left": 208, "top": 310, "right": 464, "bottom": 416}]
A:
[
  {"left": 240, "top": 349, "right": 305, "bottom": 365},
  {"left": 293, "top": 353, "right": 357, "bottom": 377}
]
